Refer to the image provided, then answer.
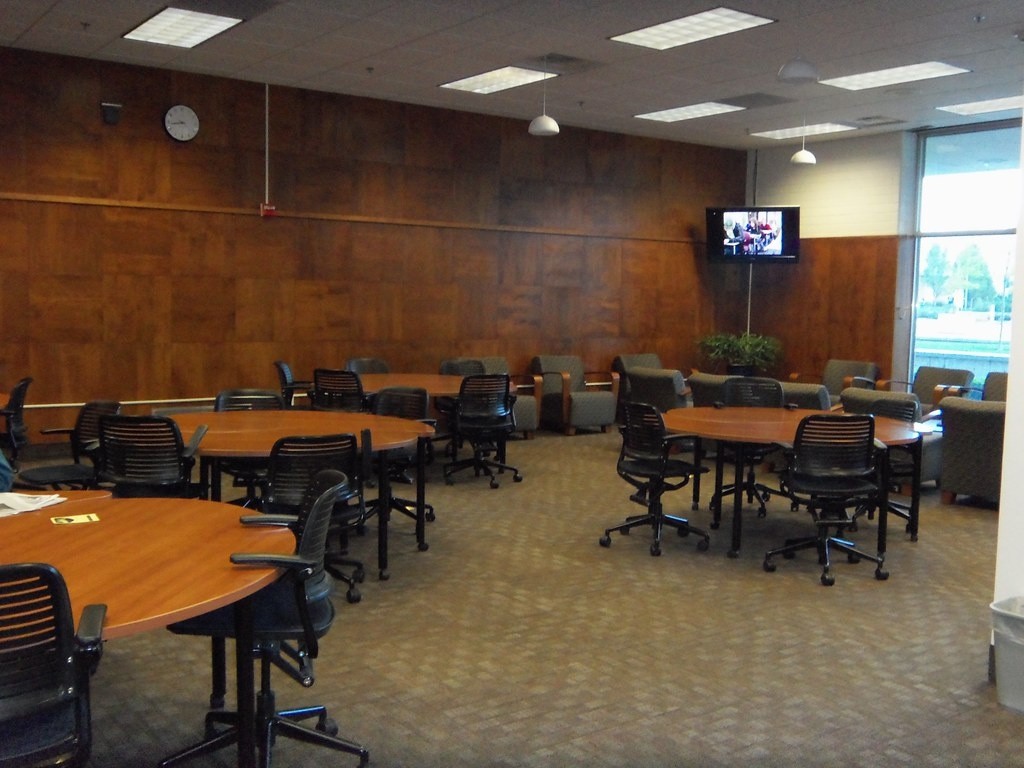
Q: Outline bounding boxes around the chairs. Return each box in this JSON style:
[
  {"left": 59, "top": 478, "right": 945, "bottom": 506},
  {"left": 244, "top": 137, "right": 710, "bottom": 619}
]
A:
[{"left": 0, "top": 353, "right": 1010, "bottom": 768}]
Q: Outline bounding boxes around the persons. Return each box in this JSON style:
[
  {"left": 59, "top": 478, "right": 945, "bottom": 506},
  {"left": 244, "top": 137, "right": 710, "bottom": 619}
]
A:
[{"left": 724, "top": 217, "right": 773, "bottom": 255}]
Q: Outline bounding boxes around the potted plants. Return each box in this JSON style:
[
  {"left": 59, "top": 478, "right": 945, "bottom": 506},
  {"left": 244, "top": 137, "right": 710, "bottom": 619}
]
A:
[{"left": 702, "top": 332, "right": 784, "bottom": 378}]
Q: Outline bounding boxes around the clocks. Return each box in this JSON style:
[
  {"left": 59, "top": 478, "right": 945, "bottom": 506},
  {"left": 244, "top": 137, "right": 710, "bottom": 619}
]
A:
[{"left": 164, "top": 105, "right": 199, "bottom": 142}]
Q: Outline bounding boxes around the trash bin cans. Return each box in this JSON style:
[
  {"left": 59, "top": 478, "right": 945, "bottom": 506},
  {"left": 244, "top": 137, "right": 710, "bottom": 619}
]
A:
[{"left": 989, "top": 595, "right": 1024, "bottom": 716}]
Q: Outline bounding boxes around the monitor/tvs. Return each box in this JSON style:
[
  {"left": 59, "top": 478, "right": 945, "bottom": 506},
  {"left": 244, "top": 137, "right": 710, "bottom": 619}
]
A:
[{"left": 705, "top": 206, "right": 800, "bottom": 264}]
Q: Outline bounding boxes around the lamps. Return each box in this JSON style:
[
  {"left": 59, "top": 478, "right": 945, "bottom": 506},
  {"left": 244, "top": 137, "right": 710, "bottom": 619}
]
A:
[
  {"left": 528, "top": 53, "right": 561, "bottom": 136},
  {"left": 791, "top": 114, "right": 816, "bottom": 165}
]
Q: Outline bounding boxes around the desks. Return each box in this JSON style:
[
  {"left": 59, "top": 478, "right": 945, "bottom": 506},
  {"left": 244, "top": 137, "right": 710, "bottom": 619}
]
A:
[
  {"left": 0, "top": 489, "right": 299, "bottom": 768},
  {"left": 161, "top": 410, "right": 438, "bottom": 577},
  {"left": 663, "top": 407, "right": 923, "bottom": 558}
]
